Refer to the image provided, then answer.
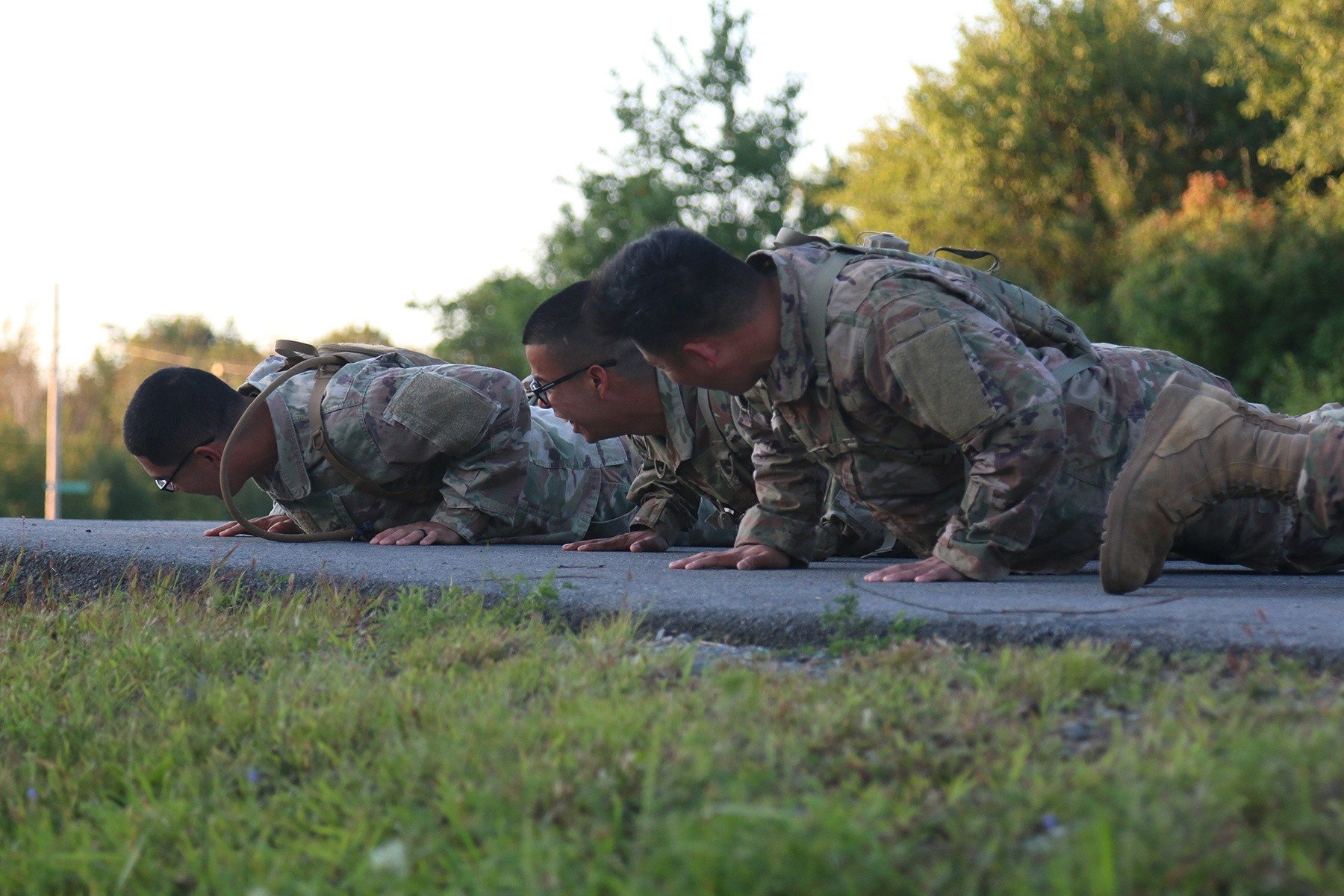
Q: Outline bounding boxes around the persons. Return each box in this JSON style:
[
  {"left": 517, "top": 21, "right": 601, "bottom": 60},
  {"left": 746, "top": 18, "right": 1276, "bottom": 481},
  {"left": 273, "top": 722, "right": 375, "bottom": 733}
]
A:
[
  {"left": 118, "top": 340, "right": 739, "bottom": 545},
  {"left": 1095, "top": 370, "right": 1344, "bottom": 596},
  {"left": 522, "top": 280, "right": 917, "bottom": 557},
  {"left": 585, "top": 225, "right": 1344, "bottom": 583}
]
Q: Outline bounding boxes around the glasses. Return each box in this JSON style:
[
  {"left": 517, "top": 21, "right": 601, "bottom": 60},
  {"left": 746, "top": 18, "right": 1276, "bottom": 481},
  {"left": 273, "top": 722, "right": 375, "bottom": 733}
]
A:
[
  {"left": 530, "top": 358, "right": 617, "bottom": 405},
  {"left": 155, "top": 437, "right": 214, "bottom": 493}
]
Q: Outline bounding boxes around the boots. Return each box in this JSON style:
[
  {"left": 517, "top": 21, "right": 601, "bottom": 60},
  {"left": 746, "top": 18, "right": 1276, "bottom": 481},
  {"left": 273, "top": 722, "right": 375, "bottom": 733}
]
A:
[
  {"left": 1100, "top": 383, "right": 1309, "bottom": 596},
  {"left": 1143, "top": 371, "right": 1302, "bottom": 584}
]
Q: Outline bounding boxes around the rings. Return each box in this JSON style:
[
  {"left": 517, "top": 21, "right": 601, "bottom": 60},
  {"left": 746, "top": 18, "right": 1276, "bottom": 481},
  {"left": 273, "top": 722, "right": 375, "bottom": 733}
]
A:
[{"left": 418, "top": 528, "right": 427, "bottom": 537}]
